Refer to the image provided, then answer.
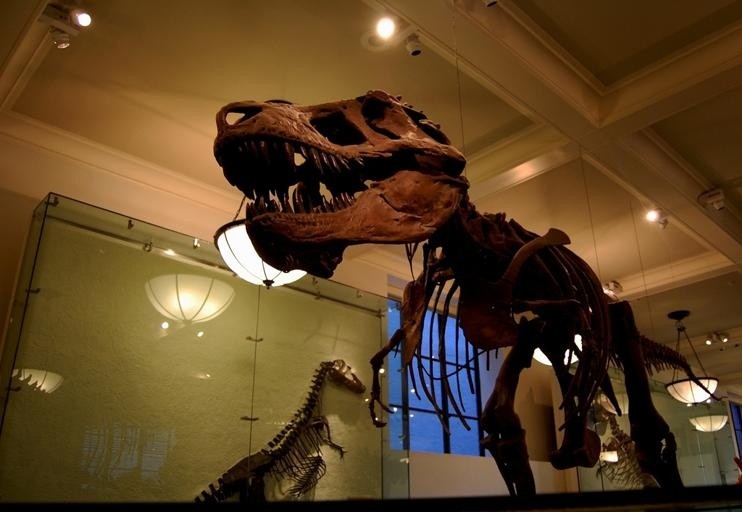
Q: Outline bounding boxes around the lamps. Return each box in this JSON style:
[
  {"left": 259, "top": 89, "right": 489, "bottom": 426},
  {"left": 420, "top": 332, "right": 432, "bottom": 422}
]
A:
[
  {"left": 146, "top": 258, "right": 239, "bottom": 326},
  {"left": 639, "top": 208, "right": 671, "bottom": 232},
  {"left": 688, "top": 408, "right": 730, "bottom": 433},
  {"left": 39, "top": 2, "right": 95, "bottom": 51},
  {"left": 661, "top": 307, "right": 718, "bottom": 406},
  {"left": 701, "top": 331, "right": 729, "bottom": 346},
  {"left": 213, "top": 98, "right": 311, "bottom": 287},
  {"left": 360, "top": 12, "right": 426, "bottom": 62},
  {"left": 599, "top": 392, "right": 630, "bottom": 415},
  {"left": 13, "top": 346, "right": 72, "bottom": 397}
]
